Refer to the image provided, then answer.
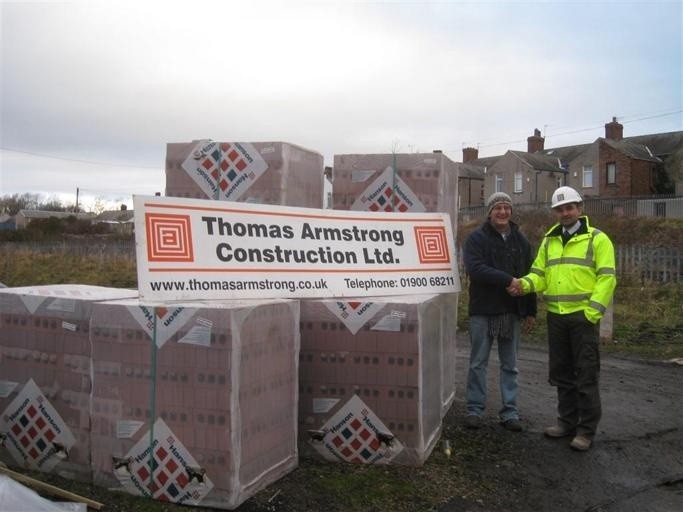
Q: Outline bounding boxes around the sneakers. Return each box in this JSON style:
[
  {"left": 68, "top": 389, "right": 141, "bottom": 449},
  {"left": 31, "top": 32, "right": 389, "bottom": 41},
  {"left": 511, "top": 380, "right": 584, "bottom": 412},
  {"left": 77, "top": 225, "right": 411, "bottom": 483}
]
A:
[
  {"left": 499, "top": 418, "right": 523, "bottom": 433},
  {"left": 567, "top": 433, "right": 592, "bottom": 453},
  {"left": 542, "top": 416, "right": 576, "bottom": 438},
  {"left": 463, "top": 413, "right": 481, "bottom": 429}
]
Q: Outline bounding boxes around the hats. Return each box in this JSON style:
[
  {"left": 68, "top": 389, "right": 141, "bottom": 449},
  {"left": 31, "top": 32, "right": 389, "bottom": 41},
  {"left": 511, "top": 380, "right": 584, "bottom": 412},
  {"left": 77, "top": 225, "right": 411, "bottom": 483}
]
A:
[{"left": 485, "top": 190, "right": 513, "bottom": 218}]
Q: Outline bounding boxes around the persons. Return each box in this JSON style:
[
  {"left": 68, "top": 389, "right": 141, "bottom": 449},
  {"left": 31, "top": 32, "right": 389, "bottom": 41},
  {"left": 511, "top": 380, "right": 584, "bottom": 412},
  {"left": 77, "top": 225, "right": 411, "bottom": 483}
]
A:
[
  {"left": 505, "top": 187, "right": 619, "bottom": 452},
  {"left": 459, "top": 190, "right": 538, "bottom": 433}
]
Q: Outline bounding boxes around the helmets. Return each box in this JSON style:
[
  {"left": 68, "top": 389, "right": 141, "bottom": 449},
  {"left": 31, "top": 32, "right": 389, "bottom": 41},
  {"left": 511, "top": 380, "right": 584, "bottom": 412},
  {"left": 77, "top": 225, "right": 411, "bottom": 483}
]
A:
[{"left": 549, "top": 185, "right": 583, "bottom": 209}]
